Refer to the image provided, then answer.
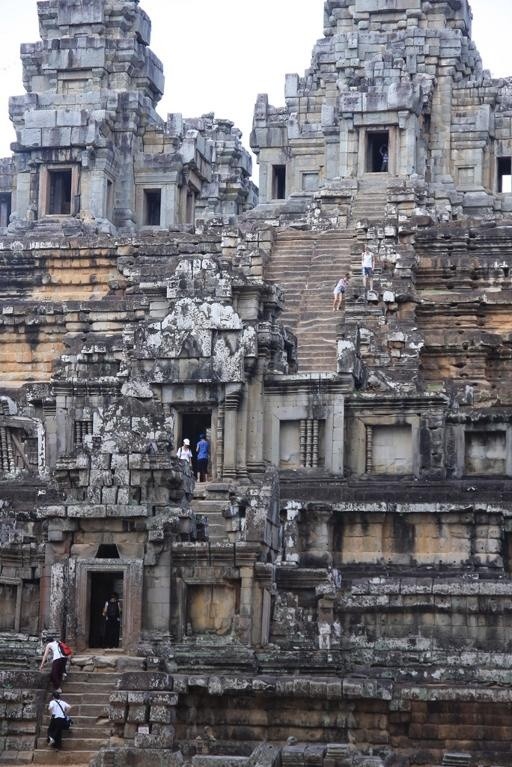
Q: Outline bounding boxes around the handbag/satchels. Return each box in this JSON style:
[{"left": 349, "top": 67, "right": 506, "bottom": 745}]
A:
[
  {"left": 59, "top": 643, "right": 71, "bottom": 656},
  {"left": 64, "top": 718, "right": 72, "bottom": 729}
]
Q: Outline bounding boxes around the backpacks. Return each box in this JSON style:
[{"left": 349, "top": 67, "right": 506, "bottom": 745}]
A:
[{"left": 107, "top": 600, "right": 119, "bottom": 620}]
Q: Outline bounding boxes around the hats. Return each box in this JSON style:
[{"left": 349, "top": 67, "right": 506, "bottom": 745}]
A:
[
  {"left": 184, "top": 439, "right": 190, "bottom": 445},
  {"left": 200, "top": 434, "right": 205, "bottom": 438}
]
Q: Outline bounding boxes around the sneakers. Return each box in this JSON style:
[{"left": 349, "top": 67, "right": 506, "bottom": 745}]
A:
[
  {"left": 55, "top": 687, "right": 62, "bottom": 694},
  {"left": 62, "top": 672, "right": 68, "bottom": 681}
]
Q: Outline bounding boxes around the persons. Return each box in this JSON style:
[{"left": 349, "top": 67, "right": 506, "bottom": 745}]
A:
[
  {"left": 175, "top": 437, "right": 193, "bottom": 467},
  {"left": 360, "top": 246, "right": 377, "bottom": 293},
  {"left": 100, "top": 592, "right": 121, "bottom": 649},
  {"left": 48, "top": 690, "right": 73, "bottom": 747},
  {"left": 333, "top": 273, "right": 351, "bottom": 310},
  {"left": 194, "top": 433, "right": 210, "bottom": 480},
  {"left": 378, "top": 142, "right": 388, "bottom": 171},
  {"left": 39, "top": 635, "right": 69, "bottom": 693}
]
